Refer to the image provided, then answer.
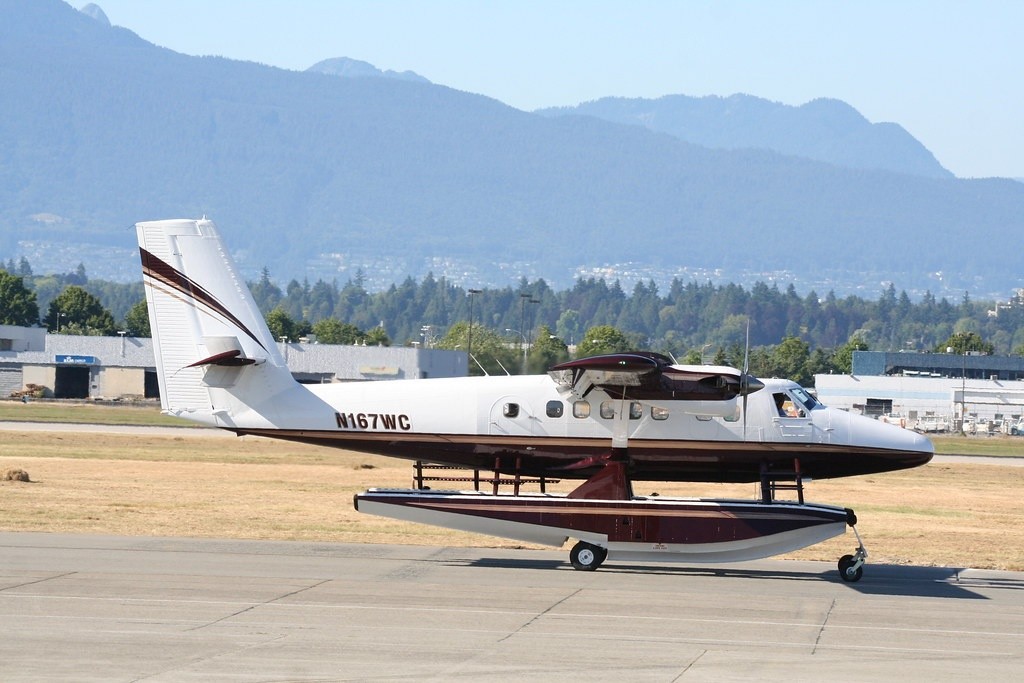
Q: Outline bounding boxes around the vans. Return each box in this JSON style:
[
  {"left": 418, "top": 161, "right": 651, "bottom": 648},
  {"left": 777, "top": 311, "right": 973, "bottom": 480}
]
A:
[
  {"left": 1011, "top": 416, "right": 1024, "bottom": 436},
  {"left": 879, "top": 412, "right": 902, "bottom": 426}
]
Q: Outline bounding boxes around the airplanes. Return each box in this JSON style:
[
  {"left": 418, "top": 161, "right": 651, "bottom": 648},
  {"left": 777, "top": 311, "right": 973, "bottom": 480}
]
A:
[{"left": 133, "top": 218, "right": 933, "bottom": 582}]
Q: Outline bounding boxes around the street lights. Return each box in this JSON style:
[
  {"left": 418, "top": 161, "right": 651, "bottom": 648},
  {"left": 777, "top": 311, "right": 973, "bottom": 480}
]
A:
[
  {"left": 528, "top": 300, "right": 540, "bottom": 358},
  {"left": 468, "top": 289, "right": 482, "bottom": 354},
  {"left": 505, "top": 328, "right": 527, "bottom": 358},
  {"left": 57, "top": 313, "right": 66, "bottom": 333},
  {"left": 518, "top": 294, "right": 532, "bottom": 360}
]
